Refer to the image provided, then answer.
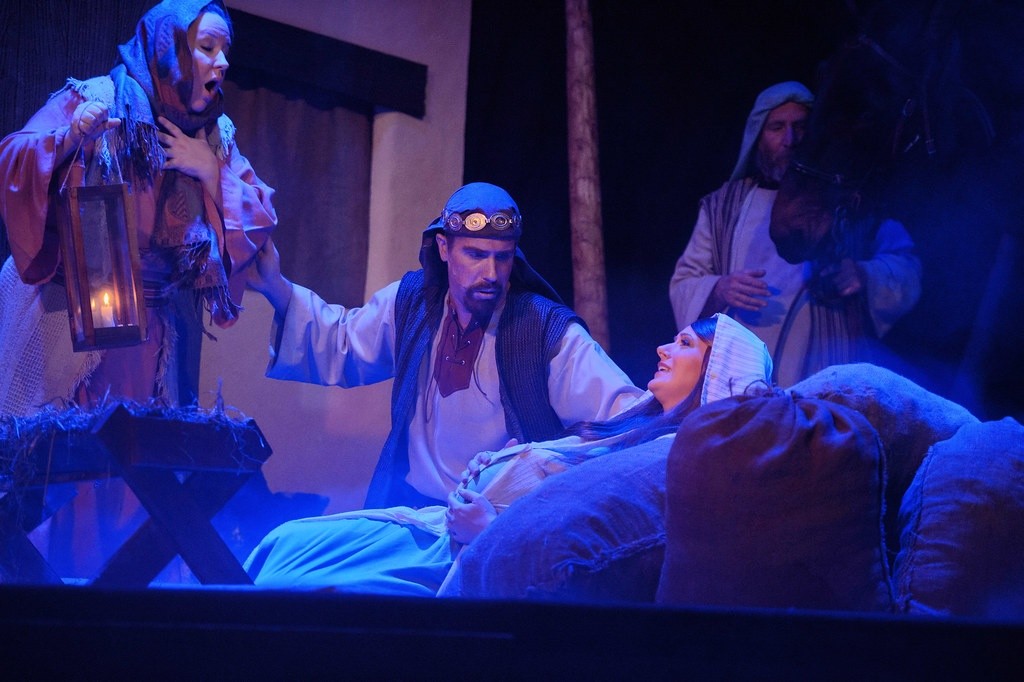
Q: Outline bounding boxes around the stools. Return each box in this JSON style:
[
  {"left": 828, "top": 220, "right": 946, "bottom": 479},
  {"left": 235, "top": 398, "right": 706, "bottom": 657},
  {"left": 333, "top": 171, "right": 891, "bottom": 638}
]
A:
[{"left": 1, "top": 383, "right": 276, "bottom": 589}]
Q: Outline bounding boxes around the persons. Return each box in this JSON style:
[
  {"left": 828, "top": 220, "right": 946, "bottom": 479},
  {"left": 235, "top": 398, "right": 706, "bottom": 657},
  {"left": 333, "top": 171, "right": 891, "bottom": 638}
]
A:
[
  {"left": 669, "top": 80, "right": 924, "bottom": 392},
  {"left": 237, "top": 181, "right": 644, "bottom": 510},
  {"left": 243, "top": 302, "right": 770, "bottom": 588},
  {"left": 0, "top": 0, "right": 278, "bottom": 570}
]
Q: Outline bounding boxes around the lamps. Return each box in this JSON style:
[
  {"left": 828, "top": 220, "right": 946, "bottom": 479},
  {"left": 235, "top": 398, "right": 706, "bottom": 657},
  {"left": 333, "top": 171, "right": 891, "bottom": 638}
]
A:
[{"left": 60, "top": 99, "right": 145, "bottom": 353}]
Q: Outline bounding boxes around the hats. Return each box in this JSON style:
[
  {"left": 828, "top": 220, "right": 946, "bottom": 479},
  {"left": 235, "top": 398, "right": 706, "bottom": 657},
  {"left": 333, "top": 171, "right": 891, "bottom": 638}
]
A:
[{"left": 417, "top": 182, "right": 566, "bottom": 320}]
